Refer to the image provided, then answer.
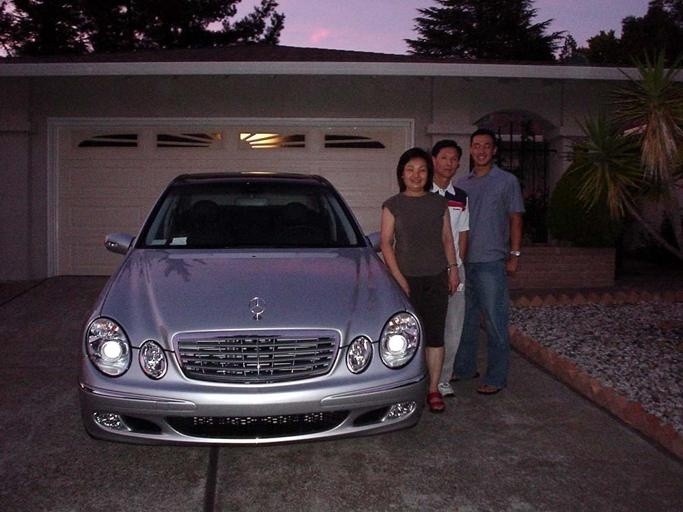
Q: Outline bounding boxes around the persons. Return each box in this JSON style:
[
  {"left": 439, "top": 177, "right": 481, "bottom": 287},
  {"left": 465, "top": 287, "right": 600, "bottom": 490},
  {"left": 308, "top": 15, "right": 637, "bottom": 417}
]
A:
[
  {"left": 450, "top": 129, "right": 525, "bottom": 394},
  {"left": 380, "top": 148, "right": 461, "bottom": 413},
  {"left": 430, "top": 140, "right": 471, "bottom": 397}
]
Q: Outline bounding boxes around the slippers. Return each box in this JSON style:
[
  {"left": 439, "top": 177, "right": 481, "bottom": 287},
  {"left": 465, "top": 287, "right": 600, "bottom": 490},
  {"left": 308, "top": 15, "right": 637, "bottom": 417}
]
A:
[
  {"left": 426, "top": 390, "right": 445, "bottom": 414},
  {"left": 476, "top": 384, "right": 499, "bottom": 394}
]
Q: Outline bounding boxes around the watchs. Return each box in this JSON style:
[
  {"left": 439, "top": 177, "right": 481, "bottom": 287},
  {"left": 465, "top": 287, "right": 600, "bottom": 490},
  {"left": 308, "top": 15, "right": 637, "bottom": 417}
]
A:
[{"left": 510, "top": 250, "right": 521, "bottom": 257}]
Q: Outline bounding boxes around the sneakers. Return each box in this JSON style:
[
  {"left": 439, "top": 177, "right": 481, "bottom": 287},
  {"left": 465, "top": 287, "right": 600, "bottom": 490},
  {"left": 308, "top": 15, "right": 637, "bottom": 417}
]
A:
[{"left": 438, "top": 382, "right": 455, "bottom": 396}]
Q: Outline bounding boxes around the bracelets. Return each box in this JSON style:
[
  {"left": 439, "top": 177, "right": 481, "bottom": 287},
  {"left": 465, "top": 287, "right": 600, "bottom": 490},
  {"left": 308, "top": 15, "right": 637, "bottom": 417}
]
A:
[{"left": 448, "top": 263, "right": 457, "bottom": 268}]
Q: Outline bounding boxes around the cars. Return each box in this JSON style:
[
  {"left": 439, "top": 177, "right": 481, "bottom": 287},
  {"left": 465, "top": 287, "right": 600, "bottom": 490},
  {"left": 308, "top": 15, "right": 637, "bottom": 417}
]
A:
[{"left": 81, "top": 173, "right": 426, "bottom": 447}]
